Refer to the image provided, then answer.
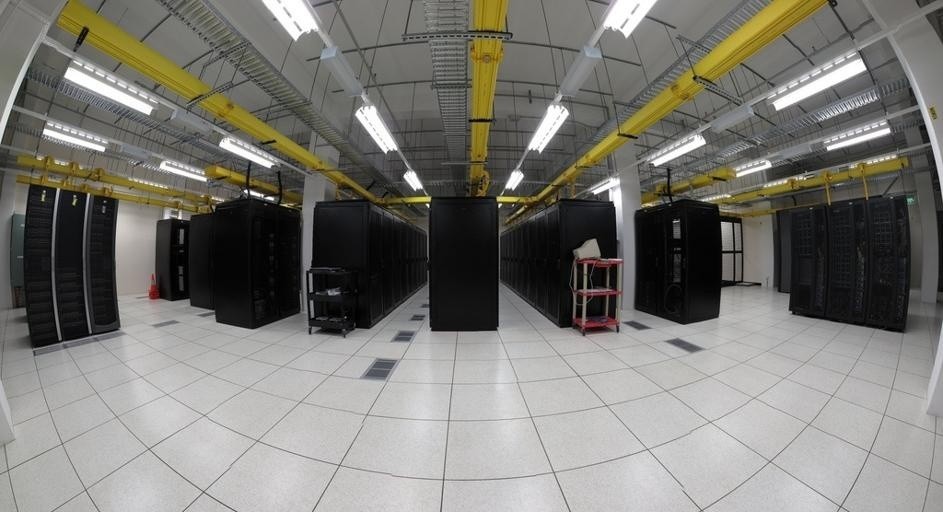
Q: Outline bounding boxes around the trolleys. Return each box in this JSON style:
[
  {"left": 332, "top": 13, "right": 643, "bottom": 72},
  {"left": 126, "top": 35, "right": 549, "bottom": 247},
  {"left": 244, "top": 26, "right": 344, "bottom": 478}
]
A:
[
  {"left": 569, "top": 254, "right": 622, "bottom": 336},
  {"left": 305, "top": 265, "right": 358, "bottom": 338}
]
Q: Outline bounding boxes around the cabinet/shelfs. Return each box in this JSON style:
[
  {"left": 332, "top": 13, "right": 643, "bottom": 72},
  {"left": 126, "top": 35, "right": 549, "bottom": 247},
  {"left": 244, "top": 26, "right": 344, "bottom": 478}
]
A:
[
  {"left": 772, "top": 190, "right": 916, "bottom": 335},
  {"left": 6, "top": 183, "right": 123, "bottom": 357},
  {"left": 154, "top": 200, "right": 302, "bottom": 331},
  {"left": 632, "top": 195, "right": 725, "bottom": 329}
]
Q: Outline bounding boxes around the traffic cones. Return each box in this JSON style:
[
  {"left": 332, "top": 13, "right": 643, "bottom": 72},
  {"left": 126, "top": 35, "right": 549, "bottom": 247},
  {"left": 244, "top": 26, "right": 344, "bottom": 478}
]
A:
[{"left": 148, "top": 272, "right": 160, "bottom": 300}]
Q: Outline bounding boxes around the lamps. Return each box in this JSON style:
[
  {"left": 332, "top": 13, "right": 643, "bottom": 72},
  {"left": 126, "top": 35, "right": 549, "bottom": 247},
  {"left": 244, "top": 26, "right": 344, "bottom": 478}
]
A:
[
  {"left": 756, "top": 46, "right": 872, "bottom": 114},
  {"left": 602, "top": 1, "right": 656, "bottom": 42},
  {"left": 349, "top": 101, "right": 438, "bottom": 210},
  {"left": 495, "top": 102, "right": 570, "bottom": 209},
  {"left": 42, "top": 61, "right": 280, "bottom": 202},
  {"left": 253, "top": 0, "right": 323, "bottom": 46}
]
